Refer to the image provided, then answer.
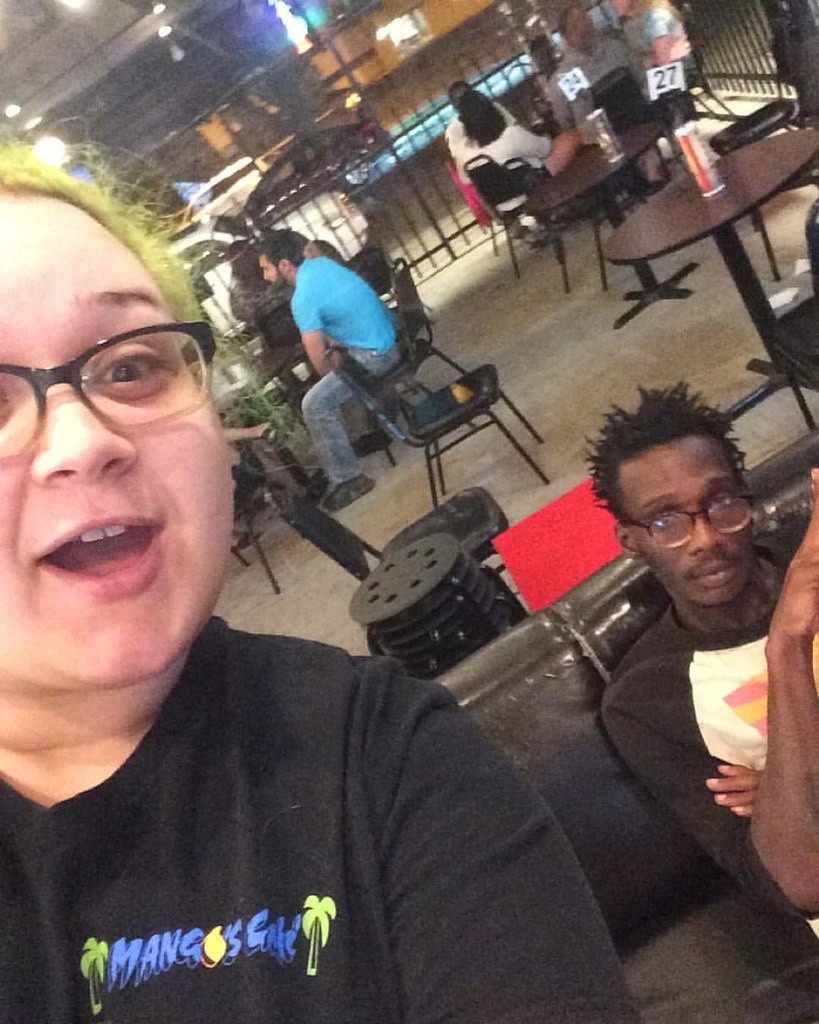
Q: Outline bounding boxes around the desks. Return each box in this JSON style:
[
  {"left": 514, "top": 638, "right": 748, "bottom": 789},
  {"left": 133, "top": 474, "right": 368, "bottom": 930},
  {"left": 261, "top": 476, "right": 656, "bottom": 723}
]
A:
[
  {"left": 527, "top": 120, "right": 699, "bottom": 326},
  {"left": 601, "top": 130, "right": 819, "bottom": 432}
]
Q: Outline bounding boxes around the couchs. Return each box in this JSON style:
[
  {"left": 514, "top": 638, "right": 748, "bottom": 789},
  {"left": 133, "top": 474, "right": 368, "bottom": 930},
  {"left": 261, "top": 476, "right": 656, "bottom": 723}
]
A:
[{"left": 438, "top": 444, "right": 819, "bottom": 1024}]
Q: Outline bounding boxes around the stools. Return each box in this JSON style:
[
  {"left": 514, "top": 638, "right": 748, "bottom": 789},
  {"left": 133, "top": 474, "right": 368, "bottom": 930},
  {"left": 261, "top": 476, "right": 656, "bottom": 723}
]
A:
[{"left": 350, "top": 533, "right": 525, "bottom": 683}]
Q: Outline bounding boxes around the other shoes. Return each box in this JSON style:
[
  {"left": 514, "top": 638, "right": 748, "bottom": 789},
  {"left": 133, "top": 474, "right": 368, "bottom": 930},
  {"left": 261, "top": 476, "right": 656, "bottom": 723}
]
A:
[{"left": 321, "top": 474, "right": 378, "bottom": 513}]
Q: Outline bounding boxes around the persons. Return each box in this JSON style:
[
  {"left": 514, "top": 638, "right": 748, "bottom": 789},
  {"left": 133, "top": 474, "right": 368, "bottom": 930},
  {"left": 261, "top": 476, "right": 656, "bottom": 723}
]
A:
[
  {"left": 0, "top": 125, "right": 645, "bottom": 1024},
  {"left": 582, "top": 381, "right": 819, "bottom": 920},
  {"left": 257, "top": 227, "right": 410, "bottom": 511},
  {"left": 443, "top": 0, "right": 698, "bottom": 256}
]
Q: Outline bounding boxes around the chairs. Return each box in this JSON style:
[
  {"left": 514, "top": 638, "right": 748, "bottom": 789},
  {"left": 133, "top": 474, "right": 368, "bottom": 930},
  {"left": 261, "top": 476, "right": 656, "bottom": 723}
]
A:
[{"left": 228, "top": 4, "right": 819, "bottom": 581}]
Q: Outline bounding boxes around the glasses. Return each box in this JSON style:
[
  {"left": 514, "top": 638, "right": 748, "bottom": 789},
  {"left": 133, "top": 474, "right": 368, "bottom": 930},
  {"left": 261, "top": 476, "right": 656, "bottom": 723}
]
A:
[
  {"left": 620, "top": 488, "right": 760, "bottom": 550},
  {"left": 0, "top": 321, "right": 220, "bottom": 460}
]
